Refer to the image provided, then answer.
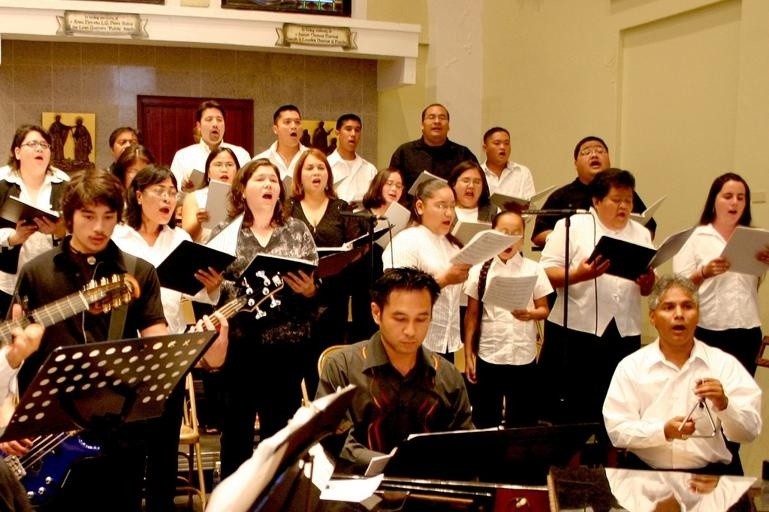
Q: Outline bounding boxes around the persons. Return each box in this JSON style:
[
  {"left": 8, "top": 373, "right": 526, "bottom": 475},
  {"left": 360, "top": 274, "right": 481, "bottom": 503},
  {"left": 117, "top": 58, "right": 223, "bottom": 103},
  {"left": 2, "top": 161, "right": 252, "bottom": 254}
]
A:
[{"left": 1, "top": 103, "right": 768, "bottom": 512}]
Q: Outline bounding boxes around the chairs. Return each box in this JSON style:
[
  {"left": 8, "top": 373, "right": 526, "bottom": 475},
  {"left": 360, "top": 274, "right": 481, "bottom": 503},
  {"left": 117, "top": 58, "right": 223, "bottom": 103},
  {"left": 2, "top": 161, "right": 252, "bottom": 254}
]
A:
[
  {"left": 317, "top": 344, "right": 353, "bottom": 379},
  {"left": 142, "top": 371, "right": 207, "bottom": 512}
]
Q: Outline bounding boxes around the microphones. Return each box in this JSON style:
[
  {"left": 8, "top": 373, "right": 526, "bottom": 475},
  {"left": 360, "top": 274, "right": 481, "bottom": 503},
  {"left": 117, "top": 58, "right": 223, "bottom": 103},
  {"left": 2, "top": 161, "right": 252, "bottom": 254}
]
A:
[
  {"left": 520, "top": 208, "right": 577, "bottom": 217},
  {"left": 339, "top": 211, "right": 387, "bottom": 220}
]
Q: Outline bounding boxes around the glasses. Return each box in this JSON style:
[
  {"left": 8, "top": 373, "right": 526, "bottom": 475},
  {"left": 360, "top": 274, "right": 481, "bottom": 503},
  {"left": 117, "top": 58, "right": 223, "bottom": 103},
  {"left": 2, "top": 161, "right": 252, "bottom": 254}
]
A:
[
  {"left": 20, "top": 140, "right": 53, "bottom": 150},
  {"left": 148, "top": 187, "right": 183, "bottom": 201}
]
